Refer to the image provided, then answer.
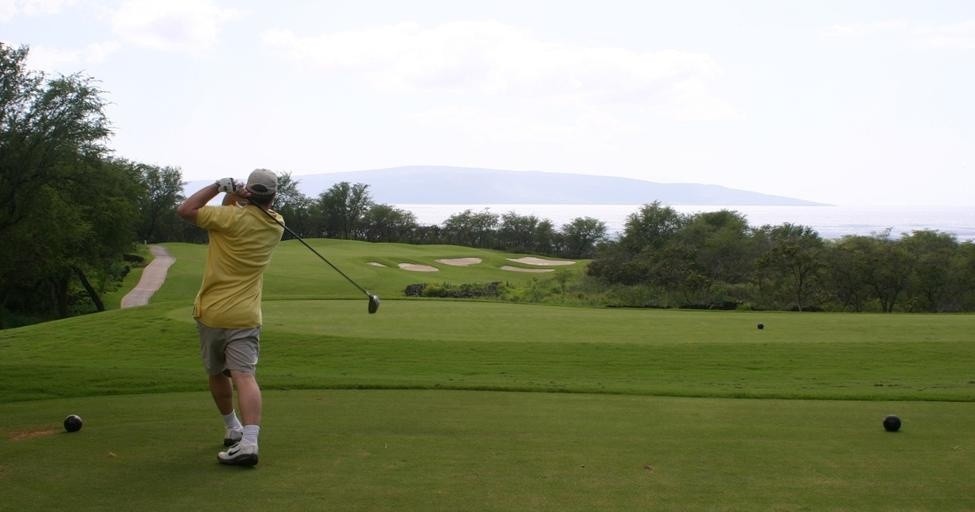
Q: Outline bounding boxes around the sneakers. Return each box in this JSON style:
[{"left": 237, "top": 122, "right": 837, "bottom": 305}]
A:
[
  {"left": 224, "top": 427, "right": 245, "bottom": 447},
  {"left": 217, "top": 442, "right": 259, "bottom": 466}
]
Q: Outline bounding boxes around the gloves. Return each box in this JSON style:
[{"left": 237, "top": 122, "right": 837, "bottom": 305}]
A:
[{"left": 216, "top": 176, "right": 245, "bottom": 193}]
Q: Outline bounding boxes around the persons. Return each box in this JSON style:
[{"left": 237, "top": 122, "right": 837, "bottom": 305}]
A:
[{"left": 176, "top": 167, "right": 288, "bottom": 466}]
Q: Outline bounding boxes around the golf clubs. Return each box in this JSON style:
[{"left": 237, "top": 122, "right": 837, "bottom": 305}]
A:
[{"left": 230, "top": 181, "right": 379, "bottom": 315}]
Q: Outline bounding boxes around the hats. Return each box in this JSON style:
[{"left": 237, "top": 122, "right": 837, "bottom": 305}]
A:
[{"left": 246, "top": 168, "right": 279, "bottom": 196}]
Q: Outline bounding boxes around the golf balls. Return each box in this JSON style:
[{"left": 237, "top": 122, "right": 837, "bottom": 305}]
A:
[
  {"left": 64, "top": 414, "right": 84, "bottom": 432},
  {"left": 882, "top": 416, "right": 901, "bottom": 432},
  {"left": 757, "top": 324, "right": 764, "bottom": 329}
]
